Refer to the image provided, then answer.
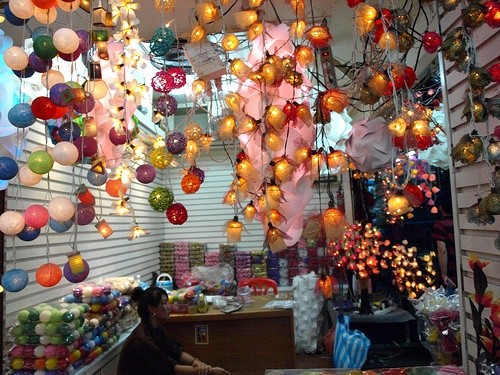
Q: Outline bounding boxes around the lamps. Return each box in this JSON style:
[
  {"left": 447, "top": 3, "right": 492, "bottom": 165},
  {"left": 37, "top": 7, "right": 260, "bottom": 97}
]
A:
[{"left": 64, "top": 0, "right": 500, "bottom": 301}]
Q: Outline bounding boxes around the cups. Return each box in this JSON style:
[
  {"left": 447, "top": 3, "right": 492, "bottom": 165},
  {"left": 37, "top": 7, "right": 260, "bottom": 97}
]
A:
[{"left": 237, "top": 286, "right": 252, "bottom": 308}]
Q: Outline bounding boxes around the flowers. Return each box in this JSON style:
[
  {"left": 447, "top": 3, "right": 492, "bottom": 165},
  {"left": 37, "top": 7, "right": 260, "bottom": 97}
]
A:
[{"left": 468, "top": 253, "right": 500, "bottom": 375}]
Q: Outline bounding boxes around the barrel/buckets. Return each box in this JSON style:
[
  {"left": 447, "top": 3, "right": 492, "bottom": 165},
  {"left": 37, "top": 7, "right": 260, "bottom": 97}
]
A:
[{"left": 155, "top": 272, "right": 173, "bottom": 293}]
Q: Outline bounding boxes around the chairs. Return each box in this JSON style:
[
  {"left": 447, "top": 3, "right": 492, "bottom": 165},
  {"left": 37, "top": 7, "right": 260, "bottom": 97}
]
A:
[{"left": 239, "top": 278, "right": 278, "bottom": 295}]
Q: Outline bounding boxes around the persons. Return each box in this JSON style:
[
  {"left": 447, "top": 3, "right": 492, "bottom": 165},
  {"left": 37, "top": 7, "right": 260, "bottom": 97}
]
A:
[{"left": 117, "top": 286, "right": 231, "bottom": 375}]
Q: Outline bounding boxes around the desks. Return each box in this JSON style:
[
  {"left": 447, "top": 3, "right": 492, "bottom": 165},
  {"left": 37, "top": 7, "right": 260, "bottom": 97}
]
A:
[
  {"left": 74, "top": 316, "right": 141, "bottom": 375},
  {"left": 324, "top": 300, "right": 419, "bottom": 369},
  {"left": 164, "top": 294, "right": 298, "bottom": 375},
  {"left": 265, "top": 366, "right": 465, "bottom": 375}
]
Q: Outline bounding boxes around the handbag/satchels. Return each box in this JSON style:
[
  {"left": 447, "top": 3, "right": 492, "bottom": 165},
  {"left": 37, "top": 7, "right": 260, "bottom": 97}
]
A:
[{"left": 333, "top": 315, "right": 370, "bottom": 369}]
